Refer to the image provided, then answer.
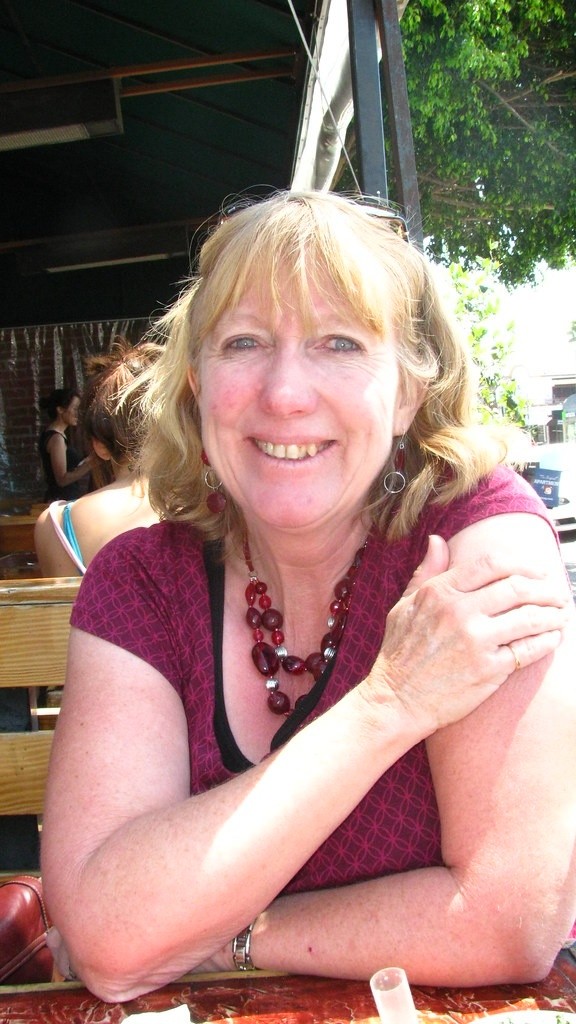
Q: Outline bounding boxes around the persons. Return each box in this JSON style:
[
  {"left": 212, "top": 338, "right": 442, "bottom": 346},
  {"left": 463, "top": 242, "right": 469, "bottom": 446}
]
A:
[
  {"left": 41, "top": 191, "right": 576, "bottom": 1005},
  {"left": 33, "top": 342, "right": 184, "bottom": 576}
]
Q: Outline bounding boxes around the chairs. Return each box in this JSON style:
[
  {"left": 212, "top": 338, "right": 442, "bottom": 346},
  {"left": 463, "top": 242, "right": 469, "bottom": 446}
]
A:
[{"left": 0, "top": 575, "right": 82, "bottom": 816}]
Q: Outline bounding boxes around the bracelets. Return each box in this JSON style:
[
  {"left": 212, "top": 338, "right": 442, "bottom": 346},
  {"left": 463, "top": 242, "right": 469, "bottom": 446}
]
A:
[{"left": 232, "top": 920, "right": 256, "bottom": 971}]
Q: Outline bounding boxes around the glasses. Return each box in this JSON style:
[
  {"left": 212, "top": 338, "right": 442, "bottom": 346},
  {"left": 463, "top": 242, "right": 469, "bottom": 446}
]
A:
[{"left": 219, "top": 200, "right": 409, "bottom": 243}]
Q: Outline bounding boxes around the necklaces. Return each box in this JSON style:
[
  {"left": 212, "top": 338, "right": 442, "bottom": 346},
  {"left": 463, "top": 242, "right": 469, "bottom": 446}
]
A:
[{"left": 242, "top": 522, "right": 378, "bottom": 718}]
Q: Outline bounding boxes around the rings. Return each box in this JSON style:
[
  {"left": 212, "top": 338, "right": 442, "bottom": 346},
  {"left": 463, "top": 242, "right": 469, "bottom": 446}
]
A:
[{"left": 508, "top": 644, "right": 521, "bottom": 670}]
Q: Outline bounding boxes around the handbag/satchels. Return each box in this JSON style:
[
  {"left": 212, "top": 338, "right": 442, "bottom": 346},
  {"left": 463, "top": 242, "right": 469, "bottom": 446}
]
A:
[{"left": 0, "top": 876, "right": 58, "bottom": 986}]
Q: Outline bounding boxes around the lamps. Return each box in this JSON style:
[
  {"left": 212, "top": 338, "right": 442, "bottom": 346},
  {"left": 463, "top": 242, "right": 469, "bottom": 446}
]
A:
[
  {"left": 12, "top": 226, "right": 189, "bottom": 276},
  {"left": 0, "top": 78, "right": 124, "bottom": 152}
]
